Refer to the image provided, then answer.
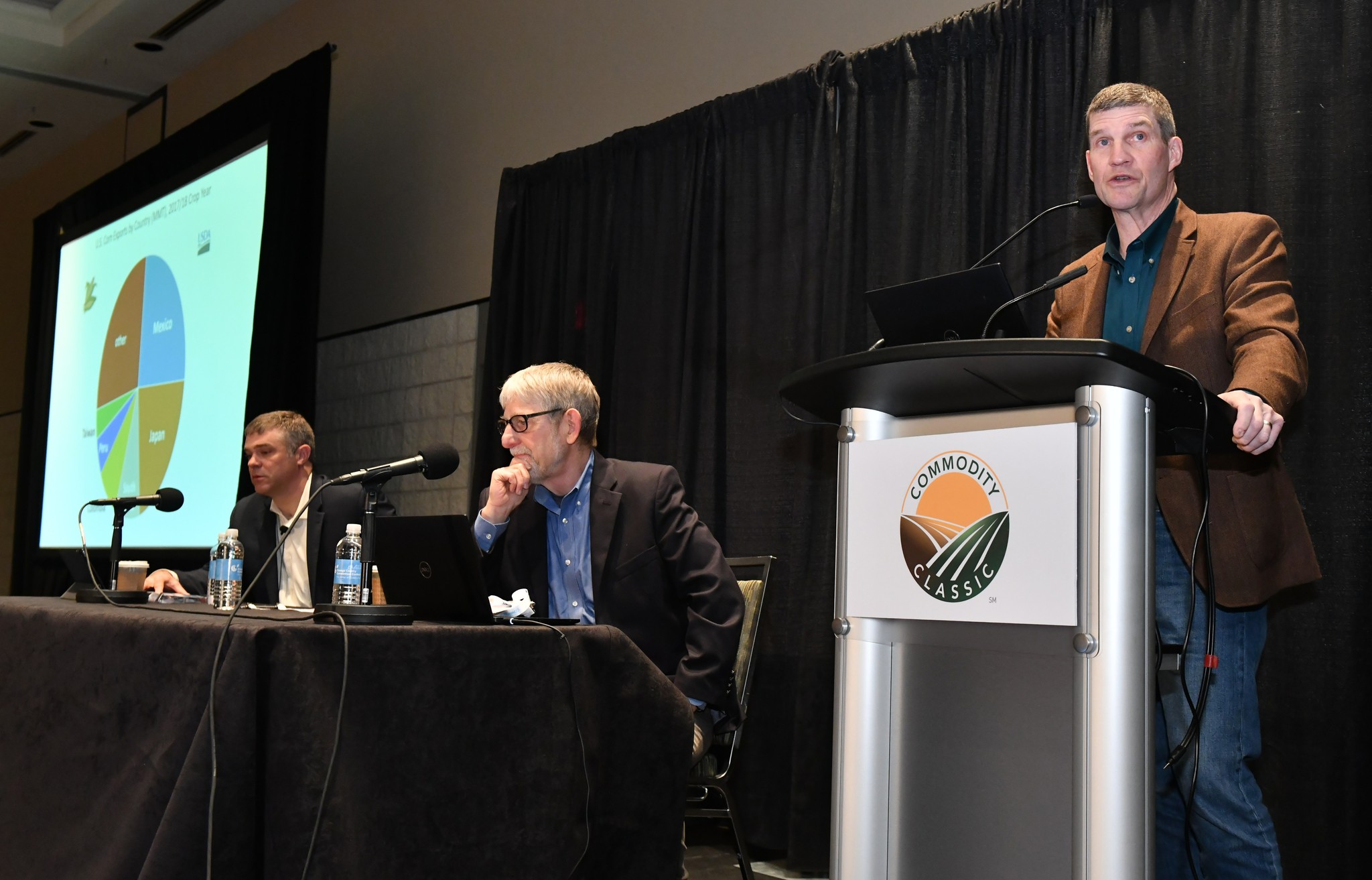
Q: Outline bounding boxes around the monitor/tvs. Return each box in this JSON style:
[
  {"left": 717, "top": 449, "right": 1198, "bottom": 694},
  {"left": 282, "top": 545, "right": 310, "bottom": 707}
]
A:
[{"left": 866, "top": 262, "right": 1029, "bottom": 348}]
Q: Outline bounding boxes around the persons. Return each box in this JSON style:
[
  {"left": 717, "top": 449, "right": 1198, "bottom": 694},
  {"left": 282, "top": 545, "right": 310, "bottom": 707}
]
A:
[
  {"left": 143, "top": 411, "right": 367, "bottom": 605},
  {"left": 1044, "top": 82, "right": 1322, "bottom": 880},
  {"left": 474, "top": 362, "right": 744, "bottom": 779}
]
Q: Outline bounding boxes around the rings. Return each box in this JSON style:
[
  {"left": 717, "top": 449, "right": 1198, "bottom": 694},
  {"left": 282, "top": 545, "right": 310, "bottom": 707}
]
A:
[{"left": 1263, "top": 420, "right": 1272, "bottom": 430}]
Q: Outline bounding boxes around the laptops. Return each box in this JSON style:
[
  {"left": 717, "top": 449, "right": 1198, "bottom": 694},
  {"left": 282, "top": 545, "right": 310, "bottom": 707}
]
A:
[{"left": 369, "top": 513, "right": 582, "bottom": 628}]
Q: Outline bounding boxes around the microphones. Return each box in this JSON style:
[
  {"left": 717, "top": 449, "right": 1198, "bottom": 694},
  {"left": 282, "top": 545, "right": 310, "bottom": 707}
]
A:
[
  {"left": 88, "top": 488, "right": 185, "bottom": 513},
  {"left": 867, "top": 194, "right": 1098, "bottom": 351},
  {"left": 981, "top": 264, "right": 1088, "bottom": 337},
  {"left": 328, "top": 442, "right": 462, "bottom": 486}
]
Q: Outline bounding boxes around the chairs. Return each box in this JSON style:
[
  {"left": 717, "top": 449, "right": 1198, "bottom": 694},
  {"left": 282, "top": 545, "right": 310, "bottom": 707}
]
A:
[{"left": 683, "top": 555, "right": 781, "bottom": 880}]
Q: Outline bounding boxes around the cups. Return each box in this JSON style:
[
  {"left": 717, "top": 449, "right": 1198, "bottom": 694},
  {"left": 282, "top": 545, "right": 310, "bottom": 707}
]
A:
[
  {"left": 372, "top": 565, "right": 386, "bottom": 605},
  {"left": 117, "top": 560, "right": 149, "bottom": 591}
]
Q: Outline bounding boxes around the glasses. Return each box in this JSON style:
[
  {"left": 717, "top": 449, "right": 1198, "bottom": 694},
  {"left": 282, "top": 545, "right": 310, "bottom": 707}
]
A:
[{"left": 496, "top": 407, "right": 563, "bottom": 435}]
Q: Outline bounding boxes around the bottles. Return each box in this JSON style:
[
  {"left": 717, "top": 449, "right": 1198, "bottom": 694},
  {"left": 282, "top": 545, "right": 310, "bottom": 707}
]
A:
[
  {"left": 213, "top": 529, "right": 244, "bottom": 611},
  {"left": 206, "top": 533, "right": 226, "bottom": 605},
  {"left": 331, "top": 524, "right": 362, "bottom": 604}
]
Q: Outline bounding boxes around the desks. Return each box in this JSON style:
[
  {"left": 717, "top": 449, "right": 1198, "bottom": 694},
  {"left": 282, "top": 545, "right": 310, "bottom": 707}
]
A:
[{"left": 0, "top": 594, "right": 697, "bottom": 880}]
